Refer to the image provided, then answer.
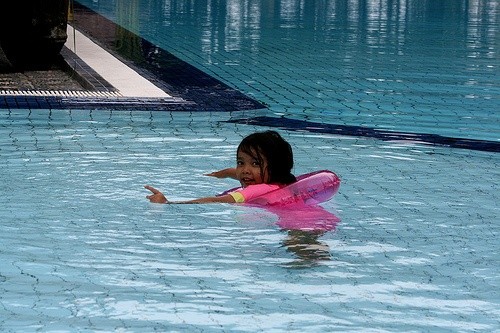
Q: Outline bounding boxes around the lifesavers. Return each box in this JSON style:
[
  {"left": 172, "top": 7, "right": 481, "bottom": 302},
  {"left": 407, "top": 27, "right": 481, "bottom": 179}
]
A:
[{"left": 216, "top": 169, "right": 340, "bottom": 207}]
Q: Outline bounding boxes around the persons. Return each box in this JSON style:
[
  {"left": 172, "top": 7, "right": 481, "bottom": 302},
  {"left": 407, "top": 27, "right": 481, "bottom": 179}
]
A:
[{"left": 144, "top": 130, "right": 339, "bottom": 269}]
